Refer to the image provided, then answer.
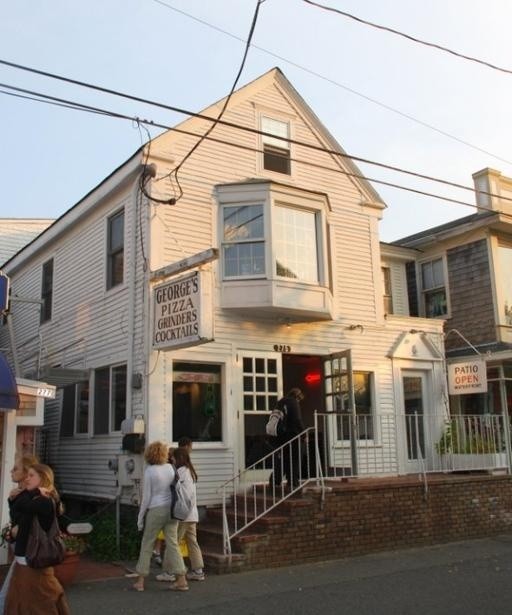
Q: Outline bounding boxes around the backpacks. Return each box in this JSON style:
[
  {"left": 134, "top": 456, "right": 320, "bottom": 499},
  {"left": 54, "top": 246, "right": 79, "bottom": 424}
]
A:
[{"left": 265, "top": 401, "right": 288, "bottom": 437}]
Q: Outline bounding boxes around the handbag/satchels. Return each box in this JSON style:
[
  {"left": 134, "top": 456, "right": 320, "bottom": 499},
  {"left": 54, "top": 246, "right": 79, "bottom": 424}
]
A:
[
  {"left": 27, "top": 516, "right": 67, "bottom": 568},
  {"left": 170, "top": 479, "right": 194, "bottom": 522}
]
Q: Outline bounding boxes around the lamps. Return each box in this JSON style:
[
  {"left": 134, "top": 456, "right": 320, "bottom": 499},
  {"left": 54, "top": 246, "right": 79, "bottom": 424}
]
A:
[{"left": 282, "top": 317, "right": 294, "bottom": 328}]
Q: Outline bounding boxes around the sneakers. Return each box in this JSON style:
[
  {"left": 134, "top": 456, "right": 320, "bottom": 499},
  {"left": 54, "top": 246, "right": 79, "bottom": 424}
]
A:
[
  {"left": 187, "top": 570, "right": 205, "bottom": 582},
  {"left": 155, "top": 555, "right": 162, "bottom": 564},
  {"left": 125, "top": 583, "right": 144, "bottom": 591},
  {"left": 154, "top": 572, "right": 176, "bottom": 582},
  {"left": 168, "top": 581, "right": 189, "bottom": 592}
]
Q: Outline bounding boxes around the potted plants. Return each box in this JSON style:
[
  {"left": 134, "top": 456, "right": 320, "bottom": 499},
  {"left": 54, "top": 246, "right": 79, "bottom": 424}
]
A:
[
  {"left": 54, "top": 535, "right": 85, "bottom": 564},
  {"left": 436, "top": 419, "right": 507, "bottom": 470}
]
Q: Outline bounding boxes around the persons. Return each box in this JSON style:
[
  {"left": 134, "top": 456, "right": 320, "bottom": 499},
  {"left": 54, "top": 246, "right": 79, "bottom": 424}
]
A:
[
  {"left": 268, "top": 387, "right": 308, "bottom": 492},
  {"left": 0, "top": 454, "right": 70, "bottom": 615},
  {"left": 128, "top": 438, "right": 205, "bottom": 592}
]
